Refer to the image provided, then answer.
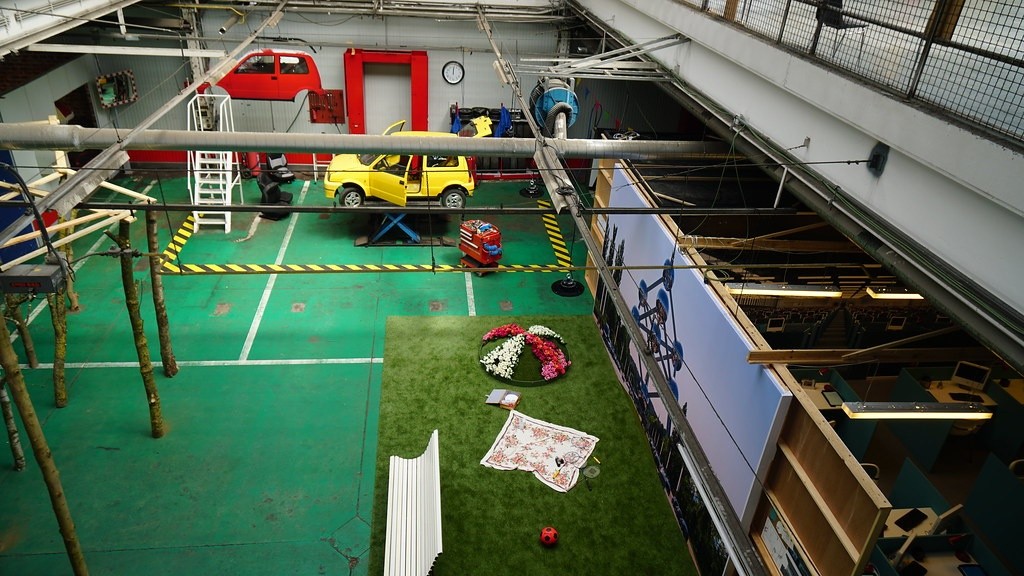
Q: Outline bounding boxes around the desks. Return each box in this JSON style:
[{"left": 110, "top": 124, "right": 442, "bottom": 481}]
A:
[
  {"left": 879, "top": 501, "right": 982, "bottom": 576},
  {"left": 796, "top": 376, "right": 847, "bottom": 411},
  {"left": 920, "top": 374, "right": 1023, "bottom": 410}
]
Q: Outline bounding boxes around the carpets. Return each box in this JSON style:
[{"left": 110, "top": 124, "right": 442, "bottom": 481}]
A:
[{"left": 365, "top": 313, "right": 703, "bottom": 576}]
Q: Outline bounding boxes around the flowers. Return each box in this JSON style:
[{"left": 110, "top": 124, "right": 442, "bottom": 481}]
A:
[{"left": 480, "top": 324, "right": 572, "bottom": 383}]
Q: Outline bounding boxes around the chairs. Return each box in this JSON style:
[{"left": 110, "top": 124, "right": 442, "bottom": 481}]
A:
[
  {"left": 736, "top": 300, "right": 965, "bottom": 349},
  {"left": 804, "top": 0, "right": 870, "bottom": 61}
]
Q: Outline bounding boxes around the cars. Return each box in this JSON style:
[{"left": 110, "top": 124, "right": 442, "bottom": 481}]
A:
[{"left": 322, "top": 114, "right": 494, "bottom": 212}]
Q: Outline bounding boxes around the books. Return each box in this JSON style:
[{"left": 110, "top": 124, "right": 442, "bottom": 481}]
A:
[{"left": 484, "top": 388, "right": 522, "bottom": 412}]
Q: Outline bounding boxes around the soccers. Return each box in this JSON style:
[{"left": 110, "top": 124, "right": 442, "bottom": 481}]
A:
[{"left": 539, "top": 527, "right": 558, "bottom": 546}]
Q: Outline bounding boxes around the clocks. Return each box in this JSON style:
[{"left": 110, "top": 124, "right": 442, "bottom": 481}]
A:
[{"left": 441, "top": 61, "right": 466, "bottom": 86}]
